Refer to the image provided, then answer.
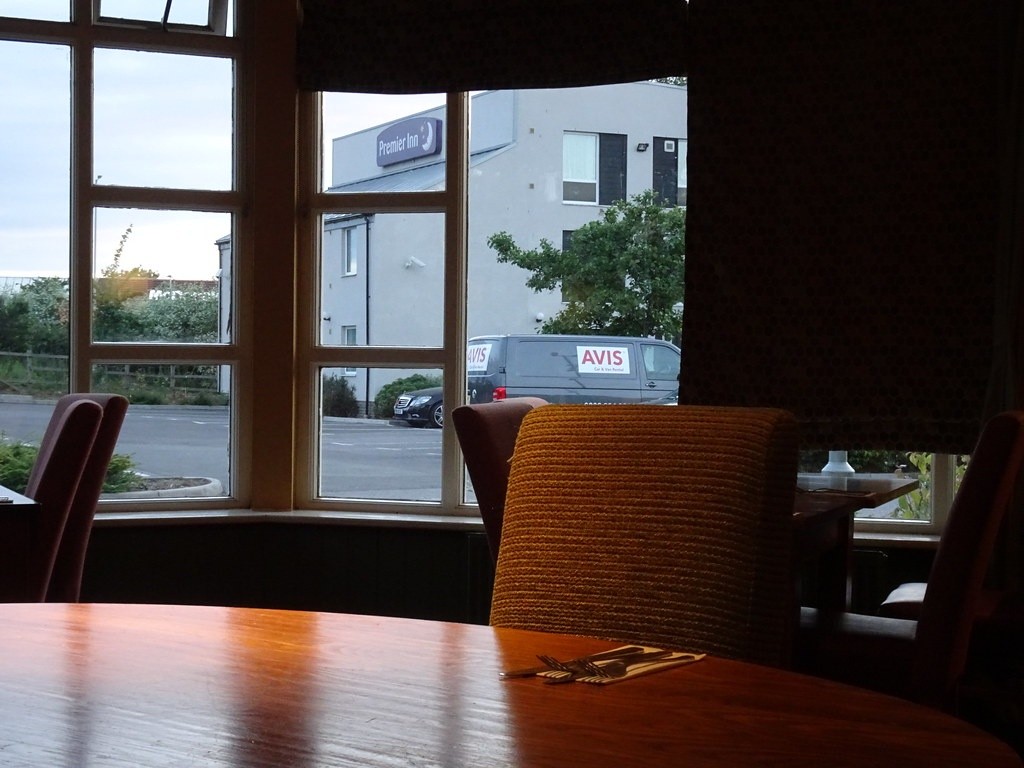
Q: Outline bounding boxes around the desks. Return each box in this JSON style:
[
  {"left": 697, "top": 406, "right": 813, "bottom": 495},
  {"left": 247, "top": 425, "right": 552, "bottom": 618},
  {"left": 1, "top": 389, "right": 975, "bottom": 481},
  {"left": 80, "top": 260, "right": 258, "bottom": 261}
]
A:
[
  {"left": 0, "top": 601, "right": 1024, "bottom": 768},
  {"left": 790, "top": 470, "right": 921, "bottom": 613},
  {"left": 0, "top": 484, "right": 41, "bottom": 545}
]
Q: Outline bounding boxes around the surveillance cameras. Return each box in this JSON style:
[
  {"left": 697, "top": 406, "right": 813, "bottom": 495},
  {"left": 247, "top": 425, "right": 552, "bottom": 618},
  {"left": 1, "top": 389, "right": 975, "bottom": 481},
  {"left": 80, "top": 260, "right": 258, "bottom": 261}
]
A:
[{"left": 411, "top": 256, "right": 426, "bottom": 267}]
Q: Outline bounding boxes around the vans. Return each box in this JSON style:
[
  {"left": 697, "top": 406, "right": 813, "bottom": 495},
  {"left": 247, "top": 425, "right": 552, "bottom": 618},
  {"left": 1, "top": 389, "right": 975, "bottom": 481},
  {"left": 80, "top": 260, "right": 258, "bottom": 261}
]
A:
[{"left": 466, "top": 333, "right": 681, "bottom": 404}]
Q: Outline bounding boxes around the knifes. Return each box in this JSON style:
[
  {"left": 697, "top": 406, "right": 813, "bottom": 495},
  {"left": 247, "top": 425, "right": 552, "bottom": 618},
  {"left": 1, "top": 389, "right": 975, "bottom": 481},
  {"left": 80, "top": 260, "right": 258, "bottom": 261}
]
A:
[{"left": 499, "top": 647, "right": 644, "bottom": 677}]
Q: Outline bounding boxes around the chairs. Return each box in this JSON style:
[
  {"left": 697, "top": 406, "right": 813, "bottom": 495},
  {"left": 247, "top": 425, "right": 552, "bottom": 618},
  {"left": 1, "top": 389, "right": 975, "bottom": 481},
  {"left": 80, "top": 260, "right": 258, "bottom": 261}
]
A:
[
  {"left": 450, "top": 402, "right": 537, "bottom": 566},
  {"left": 0, "top": 392, "right": 130, "bottom": 604},
  {"left": 489, "top": 402, "right": 803, "bottom": 676},
  {"left": 797, "top": 411, "right": 1024, "bottom": 718}
]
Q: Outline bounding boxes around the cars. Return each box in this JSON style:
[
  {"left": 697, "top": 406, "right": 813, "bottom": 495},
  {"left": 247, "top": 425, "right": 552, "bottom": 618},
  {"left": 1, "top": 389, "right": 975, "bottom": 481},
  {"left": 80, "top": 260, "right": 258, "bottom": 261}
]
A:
[{"left": 392, "top": 387, "right": 444, "bottom": 430}]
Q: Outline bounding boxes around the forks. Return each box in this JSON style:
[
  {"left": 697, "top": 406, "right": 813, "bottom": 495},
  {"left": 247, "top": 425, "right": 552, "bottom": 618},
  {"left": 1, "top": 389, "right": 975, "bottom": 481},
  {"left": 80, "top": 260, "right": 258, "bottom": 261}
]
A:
[
  {"left": 536, "top": 651, "right": 672, "bottom": 674},
  {"left": 573, "top": 655, "right": 694, "bottom": 677}
]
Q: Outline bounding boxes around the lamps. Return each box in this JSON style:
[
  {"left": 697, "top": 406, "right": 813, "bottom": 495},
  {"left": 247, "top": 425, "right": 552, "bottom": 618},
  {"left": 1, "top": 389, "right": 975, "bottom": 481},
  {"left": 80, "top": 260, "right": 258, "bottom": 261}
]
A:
[{"left": 637, "top": 143, "right": 649, "bottom": 151}]
